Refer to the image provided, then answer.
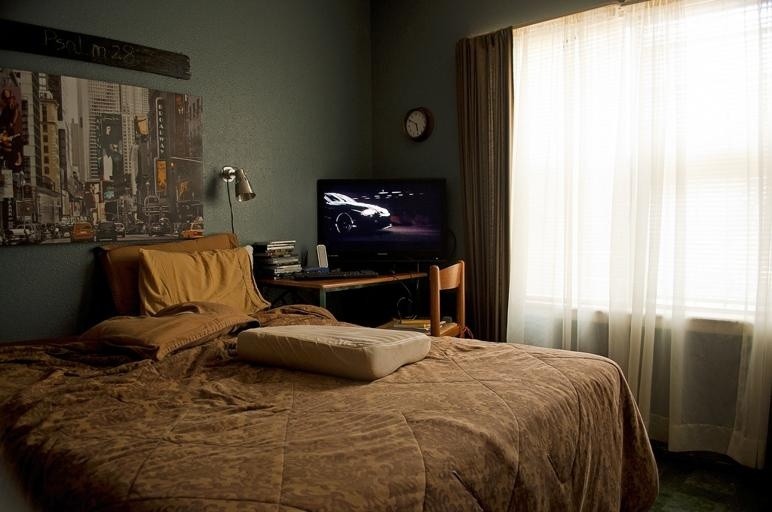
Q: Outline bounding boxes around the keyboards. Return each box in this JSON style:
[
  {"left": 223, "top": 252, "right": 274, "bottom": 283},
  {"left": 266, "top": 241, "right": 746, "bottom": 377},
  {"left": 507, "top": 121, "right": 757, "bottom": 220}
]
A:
[{"left": 291, "top": 269, "right": 377, "bottom": 279}]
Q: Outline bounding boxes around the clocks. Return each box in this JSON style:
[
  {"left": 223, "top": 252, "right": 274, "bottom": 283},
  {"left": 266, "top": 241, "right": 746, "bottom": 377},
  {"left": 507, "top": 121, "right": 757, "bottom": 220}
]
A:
[{"left": 402, "top": 107, "right": 433, "bottom": 143}]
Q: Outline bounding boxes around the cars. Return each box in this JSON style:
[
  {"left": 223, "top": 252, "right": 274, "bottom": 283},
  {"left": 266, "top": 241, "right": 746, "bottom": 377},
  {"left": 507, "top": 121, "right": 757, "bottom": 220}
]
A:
[
  {"left": 325, "top": 192, "right": 391, "bottom": 235},
  {"left": 2, "top": 212, "right": 204, "bottom": 244}
]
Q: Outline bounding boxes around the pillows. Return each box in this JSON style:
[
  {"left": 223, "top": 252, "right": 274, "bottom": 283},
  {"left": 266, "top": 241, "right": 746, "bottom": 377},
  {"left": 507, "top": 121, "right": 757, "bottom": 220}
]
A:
[
  {"left": 237, "top": 326, "right": 432, "bottom": 381},
  {"left": 138, "top": 245, "right": 271, "bottom": 318},
  {"left": 101, "top": 232, "right": 237, "bottom": 315},
  {"left": 75, "top": 301, "right": 260, "bottom": 362}
]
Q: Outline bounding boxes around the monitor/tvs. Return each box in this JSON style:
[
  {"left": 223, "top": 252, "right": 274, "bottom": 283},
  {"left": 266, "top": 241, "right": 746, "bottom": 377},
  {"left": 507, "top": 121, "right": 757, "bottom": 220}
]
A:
[{"left": 317, "top": 177, "right": 449, "bottom": 256}]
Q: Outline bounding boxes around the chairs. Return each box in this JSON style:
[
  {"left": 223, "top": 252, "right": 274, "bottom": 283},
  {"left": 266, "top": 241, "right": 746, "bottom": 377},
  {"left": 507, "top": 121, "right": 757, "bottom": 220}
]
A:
[{"left": 377, "top": 260, "right": 467, "bottom": 337}]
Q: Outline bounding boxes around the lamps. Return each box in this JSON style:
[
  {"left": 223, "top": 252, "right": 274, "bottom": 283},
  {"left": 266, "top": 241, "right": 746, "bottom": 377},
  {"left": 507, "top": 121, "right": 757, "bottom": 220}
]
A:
[{"left": 220, "top": 166, "right": 256, "bottom": 205}]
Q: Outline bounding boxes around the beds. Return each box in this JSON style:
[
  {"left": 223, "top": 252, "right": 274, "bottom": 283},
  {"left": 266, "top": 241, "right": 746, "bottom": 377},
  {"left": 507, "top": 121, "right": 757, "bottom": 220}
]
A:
[{"left": 0, "top": 239, "right": 659, "bottom": 511}]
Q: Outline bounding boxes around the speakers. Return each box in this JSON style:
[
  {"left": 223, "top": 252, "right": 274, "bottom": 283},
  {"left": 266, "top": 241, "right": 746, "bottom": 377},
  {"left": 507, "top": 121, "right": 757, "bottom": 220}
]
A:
[{"left": 316, "top": 245, "right": 329, "bottom": 267}]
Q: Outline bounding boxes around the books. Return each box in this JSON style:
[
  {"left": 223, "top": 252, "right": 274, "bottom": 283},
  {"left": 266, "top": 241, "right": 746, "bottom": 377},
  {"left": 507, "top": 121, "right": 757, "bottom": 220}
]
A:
[{"left": 251, "top": 239, "right": 302, "bottom": 279}]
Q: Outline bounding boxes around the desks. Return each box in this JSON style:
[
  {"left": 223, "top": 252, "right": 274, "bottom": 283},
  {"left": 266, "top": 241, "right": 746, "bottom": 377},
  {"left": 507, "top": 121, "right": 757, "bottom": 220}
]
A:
[{"left": 257, "top": 272, "right": 438, "bottom": 318}]
